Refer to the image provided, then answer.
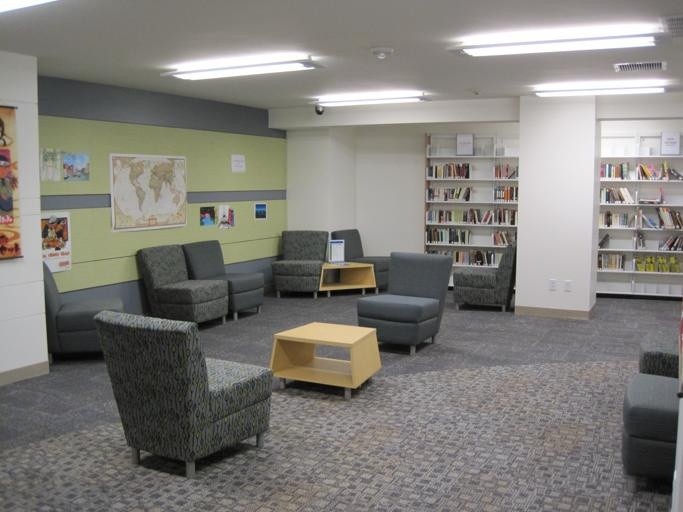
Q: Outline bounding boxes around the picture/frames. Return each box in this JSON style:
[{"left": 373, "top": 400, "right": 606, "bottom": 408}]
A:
[{"left": 455, "top": 131, "right": 475, "bottom": 157}]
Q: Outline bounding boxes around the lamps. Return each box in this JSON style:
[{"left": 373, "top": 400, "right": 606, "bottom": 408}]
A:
[
  {"left": 311, "top": 89, "right": 430, "bottom": 110},
  {"left": 536, "top": 84, "right": 672, "bottom": 104},
  {"left": 163, "top": 56, "right": 325, "bottom": 82},
  {"left": 456, "top": 34, "right": 668, "bottom": 59}
]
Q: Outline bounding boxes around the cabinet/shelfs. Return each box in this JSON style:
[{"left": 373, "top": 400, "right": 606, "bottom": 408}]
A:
[
  {"left": 423, "top": 155, "right": 518, "bottom": 289},
  {"left": 596, "top": 155, "right": 683, "bottom": 299}
]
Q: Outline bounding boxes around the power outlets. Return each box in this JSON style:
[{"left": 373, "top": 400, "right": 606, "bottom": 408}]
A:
[{"left": 548, "top": 278, "right": 558, "bottom": 291}]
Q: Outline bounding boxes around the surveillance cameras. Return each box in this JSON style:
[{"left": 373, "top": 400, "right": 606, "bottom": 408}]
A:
[{"left": 315, "top": 105, "right": 324, "bottom": 115}]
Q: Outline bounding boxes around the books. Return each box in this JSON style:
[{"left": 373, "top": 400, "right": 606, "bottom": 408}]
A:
[
  {"left": 426, "top": 207, "right": 517, "bottom": 226},
  {"left": 598, "top": 253, "right": 626, "bottom": 270},
  {"left": 493, "top": 228, "right": 516, "bottom": 246},
  {"left": 427, "top": 162, "right": 469, "bottom": 179},
  {"left": 427, "top": 186, "right": 470, "bottom": 201},
  {"left": 599, "top": 162, "right": 631, "bottom": 180},
  {"left": 599, "top": 206, "right": 635, "bottom": 227},
  {"left": 638, "top": 198, "right": 660, "bottom": 204},
  {"left": 598, "top": 234, "right": 609, "bottom": 249},
  {"left": 636, "top": 207, "right": 683, "bottom": 230},
  {"left": 636, "top": 232, "right": 683, "bottom": 252},
  {"left": 495, "top": 162, "right": 519, "bottom": 180},
  {"left": 494, "top": 185, "right": 518, "bottom": 202},
  {"left": 600, "top": 187, "right": 635, "bottom": 205},
  {"left": 658, "top": 185, "right": 664, "bottom": 204},
  {"left": 637, "top": 160, "right": 683, "bottom": 181},
  {"left": 635, "top": 255, "right": 683, "bottom": 273},
  {"left": 425, "top": 249, "right": 495, "bottom": 266},
  {"left": 425, "top": 227, "right": 471, "bottom": 245},
  {"left": 456, "top": 133, "right": 474, "bottom": 155}
]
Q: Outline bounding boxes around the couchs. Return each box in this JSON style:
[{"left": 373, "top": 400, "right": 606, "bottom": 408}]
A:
[
  {"left": 180, "top": 240, "right": 265, "bottom": 321},
  {"left": 332, "top": 230, "right": 390, "bottom": 290},
  {"left": 356, "top": 252, "right": 454, "bottom": 358},
  {"left": 453, "top": 240, "right": 516, "bottom": 313},
  {"left": 95, "top": 311, "right": 272, "bottom": 476},
  {"left": 139, "top": 245, "right": 229, "bottom": 328},
  {"left": 621, "top": 363, "right": 678, "bottom": 489},
  {"left": 270, "top": 230, "right": 328, "bottom": 298},
  {"left": 637, "top": 320, "right": 682, "bottom": 375},
  {"left": 42, "top": 260, "right": 123, "bottom": 362}
]
